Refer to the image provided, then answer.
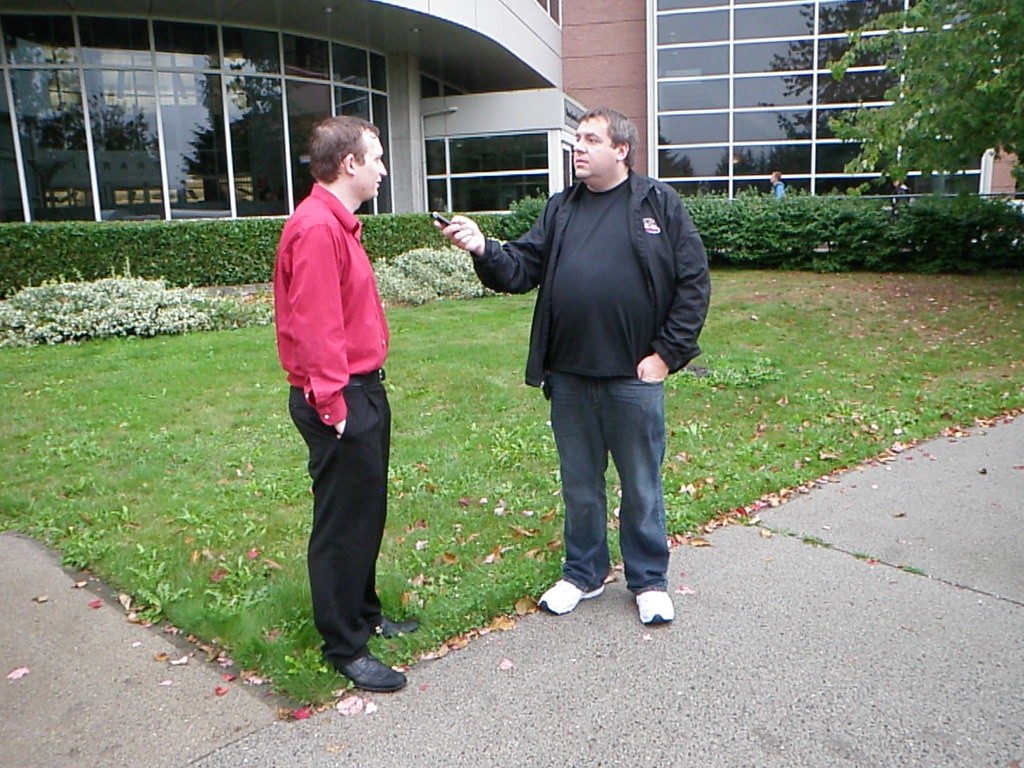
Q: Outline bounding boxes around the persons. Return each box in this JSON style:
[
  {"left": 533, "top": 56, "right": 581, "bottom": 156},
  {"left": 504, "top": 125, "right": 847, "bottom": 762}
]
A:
[
  {"left": 432, "top": 106, "right": 710, "bottom": 625},
  {"left": 273, "top": 112, "right": 421, "bottom": 694},
  {"left": 769, "top": 172, "right": 786, "bottom": 200}
]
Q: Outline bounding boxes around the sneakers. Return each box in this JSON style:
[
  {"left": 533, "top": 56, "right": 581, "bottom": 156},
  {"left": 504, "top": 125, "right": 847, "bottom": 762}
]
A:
[{"left": 536, "top": 578, "right": 675, "bottom": 625}]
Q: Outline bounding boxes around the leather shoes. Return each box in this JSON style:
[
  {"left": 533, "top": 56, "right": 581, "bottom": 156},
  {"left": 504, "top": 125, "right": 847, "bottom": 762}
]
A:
[{"left": 324, "top": 619, "right": 417, "bottom": 692}]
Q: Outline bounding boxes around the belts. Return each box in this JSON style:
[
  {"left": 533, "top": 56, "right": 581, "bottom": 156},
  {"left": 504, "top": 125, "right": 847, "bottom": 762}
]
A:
[{"left": 349, "top": 367, "right": 385, "bottom": 385}]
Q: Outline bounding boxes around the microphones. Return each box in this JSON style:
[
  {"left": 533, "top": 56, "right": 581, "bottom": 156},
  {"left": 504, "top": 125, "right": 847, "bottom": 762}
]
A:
[{"left": 430, "top": 211, "right": 453, "bottom": 227}]
